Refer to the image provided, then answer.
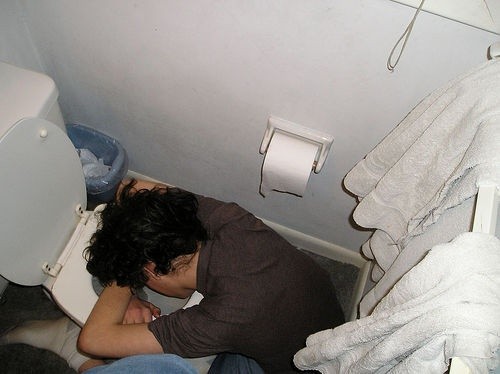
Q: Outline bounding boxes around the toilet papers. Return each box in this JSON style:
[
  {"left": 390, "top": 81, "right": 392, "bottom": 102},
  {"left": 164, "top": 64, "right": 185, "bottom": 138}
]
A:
[{"left": 260, "top": 132, "right": 318, "bottom": 199}]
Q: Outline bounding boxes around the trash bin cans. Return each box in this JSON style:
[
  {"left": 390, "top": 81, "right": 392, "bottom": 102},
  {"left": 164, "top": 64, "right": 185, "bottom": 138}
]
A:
[{"left": 65, "top": 124, "right": 129, "bottom": 210}]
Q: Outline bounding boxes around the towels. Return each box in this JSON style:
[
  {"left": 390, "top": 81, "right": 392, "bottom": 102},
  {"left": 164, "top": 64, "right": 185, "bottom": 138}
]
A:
[{"left": 293, "top": 57, "right": 500, "bottom": 373}]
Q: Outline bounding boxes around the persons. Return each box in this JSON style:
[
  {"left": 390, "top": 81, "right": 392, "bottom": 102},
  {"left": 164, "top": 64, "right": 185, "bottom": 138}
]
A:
[{"left": 0, "top": 172, "right": 348, "bottom": 374}]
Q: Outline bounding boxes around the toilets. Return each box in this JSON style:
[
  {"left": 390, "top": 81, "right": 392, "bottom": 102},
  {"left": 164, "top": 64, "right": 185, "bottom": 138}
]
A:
[{"left": 0, "top": 61, "right": 204, "bottom": 328}]
